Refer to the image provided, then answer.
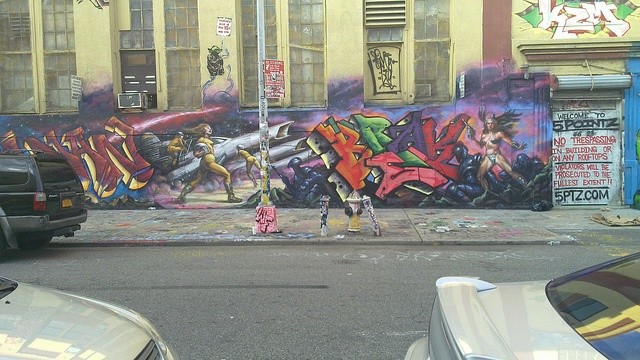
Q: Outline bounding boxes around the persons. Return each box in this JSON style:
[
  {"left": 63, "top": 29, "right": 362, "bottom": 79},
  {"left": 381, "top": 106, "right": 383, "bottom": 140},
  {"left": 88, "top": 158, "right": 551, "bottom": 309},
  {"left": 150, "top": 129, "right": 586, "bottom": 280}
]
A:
[
  {"left": 231, "top": 144, "right": 261, "bottom": 190},
  {"left": 447, "top": 144, "right": 506, "bottom": 203},
  {"left": 271, "top": 157, "right": 325, "bottom": 201},
  {"left": 498, "top": 152, "right": 545, "bottom": 181},
  {"left": 174, "top": 124, "right": 243, "bottom": 204},
  {"left": 167, "top": 132, "right": 186, "bottom": 165}
]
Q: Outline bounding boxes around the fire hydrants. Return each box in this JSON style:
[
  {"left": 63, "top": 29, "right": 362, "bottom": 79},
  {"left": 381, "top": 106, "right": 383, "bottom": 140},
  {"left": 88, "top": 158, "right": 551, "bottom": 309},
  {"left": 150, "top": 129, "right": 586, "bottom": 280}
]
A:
[{"left": 345, "top": 189, "right": 363, "bottom": 232}]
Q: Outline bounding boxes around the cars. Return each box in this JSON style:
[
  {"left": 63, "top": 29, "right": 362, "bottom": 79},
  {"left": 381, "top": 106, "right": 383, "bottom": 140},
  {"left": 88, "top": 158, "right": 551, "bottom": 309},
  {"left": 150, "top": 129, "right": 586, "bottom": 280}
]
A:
[
  {"left": 405, "top": 251, "right": 640, "bottom": 359},
  {"left": 0, "top": 275, "right": 175, "bottom": 360}
]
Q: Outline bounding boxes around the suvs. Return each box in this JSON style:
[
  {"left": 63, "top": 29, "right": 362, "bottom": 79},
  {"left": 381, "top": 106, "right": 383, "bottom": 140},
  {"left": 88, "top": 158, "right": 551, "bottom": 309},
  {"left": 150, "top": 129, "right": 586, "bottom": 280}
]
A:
[{"left": 1, "top": 151, "right": 87, "bottom": 260}]
